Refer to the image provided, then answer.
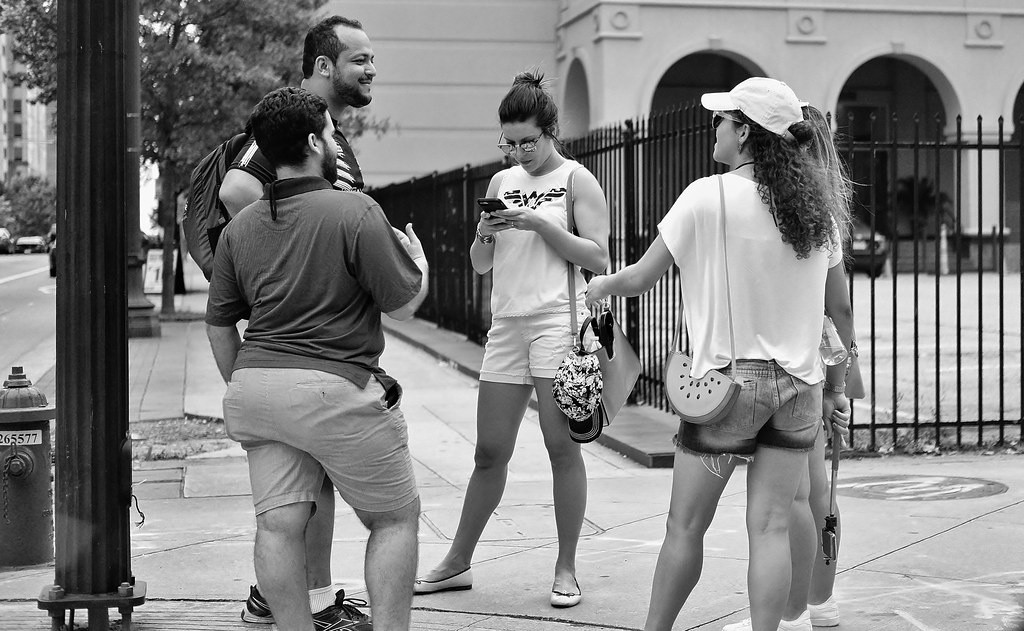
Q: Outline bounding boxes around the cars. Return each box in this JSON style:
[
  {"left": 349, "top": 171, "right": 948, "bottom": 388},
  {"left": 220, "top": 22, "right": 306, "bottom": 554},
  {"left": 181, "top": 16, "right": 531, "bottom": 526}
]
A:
[
  {"left": 844, "top": 221, "right": 889, "bottom": 278},
  {"left": 0, "top": 221, "right": 57, "bottom": 277}
]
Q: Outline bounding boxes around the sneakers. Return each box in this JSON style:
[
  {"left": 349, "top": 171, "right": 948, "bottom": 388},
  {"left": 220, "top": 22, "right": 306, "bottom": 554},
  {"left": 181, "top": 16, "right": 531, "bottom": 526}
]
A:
[
  {"left": 241, "top": 584, "right": 276, "bottom": 624},
  {"left": 722, "top": 610, "right": 813, "bottom": 631},
  {"left": 311, "top": 588, "right": 373, "bottom": 631},
  {"left": 807, "top": 595, "right": 839, "bottom": 627}
]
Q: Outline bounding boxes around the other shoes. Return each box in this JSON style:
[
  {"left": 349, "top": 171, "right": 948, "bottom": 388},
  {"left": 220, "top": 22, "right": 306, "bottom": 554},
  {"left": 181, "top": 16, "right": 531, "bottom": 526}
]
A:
[
  {"left": 550, "top": 577, "right": 582, "bottom": 607},
  {"left": 413, "top": 567, "right": 473, "bottom": 595}
]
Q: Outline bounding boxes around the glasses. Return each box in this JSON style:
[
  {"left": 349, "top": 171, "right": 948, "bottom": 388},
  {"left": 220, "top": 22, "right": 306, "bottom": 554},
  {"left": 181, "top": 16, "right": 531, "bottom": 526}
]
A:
[
  {"left": 712, "top": 111, "right": 744, "bottom": 129},
  {"left": 497, "top": 123, "right": 553, "bottom": 156}
]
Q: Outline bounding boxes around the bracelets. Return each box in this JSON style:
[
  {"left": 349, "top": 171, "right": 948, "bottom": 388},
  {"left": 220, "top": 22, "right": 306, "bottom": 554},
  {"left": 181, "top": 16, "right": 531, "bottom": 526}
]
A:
[
  {"left": 823, "top": 381, "right": 846, "bottom": 393},
  {"left": 475, "top": 221, "right": 496, "bottom": 245}
]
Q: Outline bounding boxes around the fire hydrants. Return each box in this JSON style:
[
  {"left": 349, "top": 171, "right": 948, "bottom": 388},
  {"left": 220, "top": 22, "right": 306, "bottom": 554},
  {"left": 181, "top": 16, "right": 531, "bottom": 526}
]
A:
[{"left": 0, "top": 365, "right": 56, "bottom": 572}]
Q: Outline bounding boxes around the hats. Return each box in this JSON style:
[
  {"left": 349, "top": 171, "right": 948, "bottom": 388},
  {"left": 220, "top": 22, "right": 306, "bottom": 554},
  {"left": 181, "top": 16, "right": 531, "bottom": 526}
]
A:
[
  {"left": 701, "top": 77, "right": 803, "bottom": 143},
  {"left": 553, "top": 348, "right": 603, "bottom": 421}
]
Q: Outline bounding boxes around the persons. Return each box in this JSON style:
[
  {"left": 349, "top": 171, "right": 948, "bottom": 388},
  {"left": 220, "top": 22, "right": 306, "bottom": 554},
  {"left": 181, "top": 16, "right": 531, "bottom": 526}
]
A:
[
  {"left": 583, "top": 77, "right": 851, "bottom": 631},
  {"left": 412, "top": 73, "right": 609, "bottom": 608},
  {"left": 217, "top": 16, "right": 378, "bottom": 631},
  {"left": 203, "top": 87, "right": 430, "bottom": 631},
  {"left": 720, "top": 104, "right": 866, "bottom": 631}
]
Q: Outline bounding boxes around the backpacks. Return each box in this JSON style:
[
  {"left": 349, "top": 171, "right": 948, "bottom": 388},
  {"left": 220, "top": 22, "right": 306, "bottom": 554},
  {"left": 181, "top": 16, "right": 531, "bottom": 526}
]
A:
[{"left": 181, "top": 133, "right": 251, "bottom": 282}]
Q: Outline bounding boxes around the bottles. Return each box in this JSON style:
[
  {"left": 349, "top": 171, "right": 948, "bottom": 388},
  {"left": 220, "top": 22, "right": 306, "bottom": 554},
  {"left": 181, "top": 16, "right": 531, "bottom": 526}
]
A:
[{"left": 818, "top": 309, "right": 845, "bottom": 366}]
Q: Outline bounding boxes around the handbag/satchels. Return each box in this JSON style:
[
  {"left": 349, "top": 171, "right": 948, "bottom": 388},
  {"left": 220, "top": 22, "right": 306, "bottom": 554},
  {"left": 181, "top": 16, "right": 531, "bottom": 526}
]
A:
[
  {"left": 664, "top": 351, "right": 741, "bottom": 425},
  {"left": 566, "top": 308, "right": 642, "bottom": 443}
]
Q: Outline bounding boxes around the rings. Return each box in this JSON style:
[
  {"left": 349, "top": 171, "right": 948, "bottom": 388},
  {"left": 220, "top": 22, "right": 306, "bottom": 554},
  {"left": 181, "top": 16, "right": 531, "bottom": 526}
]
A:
[
  {"left": 511, "top": 221, "right": 516, "bottom": 228},
  {"left": 585, "top": 291, "right": 587, "bottom": 295}
]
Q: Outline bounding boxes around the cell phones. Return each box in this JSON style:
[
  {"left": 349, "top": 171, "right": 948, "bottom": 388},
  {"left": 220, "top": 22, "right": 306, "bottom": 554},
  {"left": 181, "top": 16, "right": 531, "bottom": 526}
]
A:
[
  {"left": 478, "top": 197, "right": 509, "bottom": 226},
  {"left": 821, "top": 529, "right": 837, "bottom": 562}
]
